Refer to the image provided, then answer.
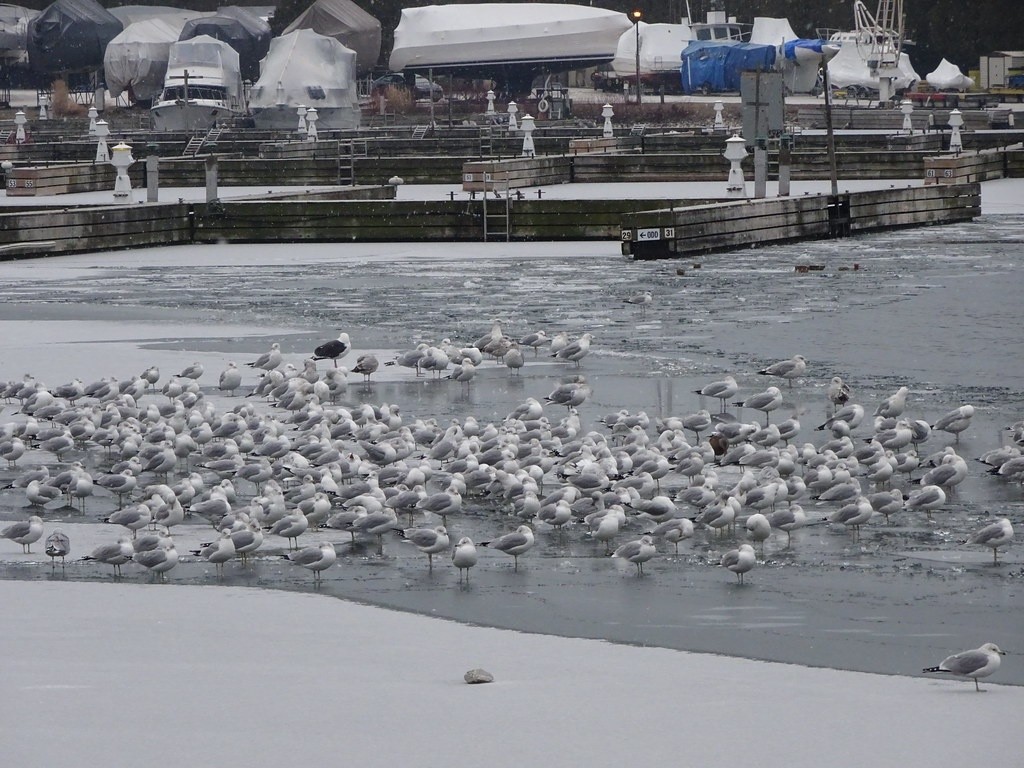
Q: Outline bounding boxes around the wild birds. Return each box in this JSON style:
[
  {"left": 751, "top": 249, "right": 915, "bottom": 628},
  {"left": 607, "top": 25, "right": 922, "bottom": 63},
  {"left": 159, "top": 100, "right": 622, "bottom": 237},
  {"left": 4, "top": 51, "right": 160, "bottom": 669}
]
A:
[
  {"left": 922, "top": 642, "right": 1007, "bottom": 693},
  {"left": 622, "top": 291, "right": 653, "bottom": 319},
  {"left": 1, "top": 320, "right": 1024, "bottom": 587}
]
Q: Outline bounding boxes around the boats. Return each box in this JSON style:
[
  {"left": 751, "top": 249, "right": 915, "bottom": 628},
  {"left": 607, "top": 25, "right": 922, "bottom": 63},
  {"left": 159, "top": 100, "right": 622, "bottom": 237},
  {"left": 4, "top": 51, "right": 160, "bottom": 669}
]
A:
[
  {"left": 600, "top": 0, "right": 974, "bottom": 103},
  {"left": 0, "top": 1, "right": 278, "bottom": 126},
  {"left": 387, "top": 3, "right": 635, "bottom": 105},
  {"left": 280, "top": 0, "right": 382, "bottom": 93},
  {"left": 150, "top": 33, "right": 248, "bottom": 136},
  {"left": 243, "top": 27, "right": 362, "bottom": 134}
]
